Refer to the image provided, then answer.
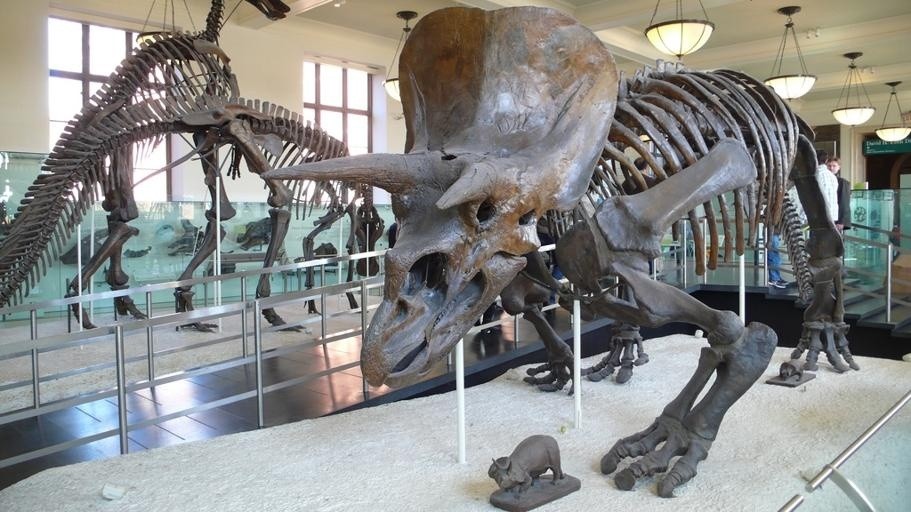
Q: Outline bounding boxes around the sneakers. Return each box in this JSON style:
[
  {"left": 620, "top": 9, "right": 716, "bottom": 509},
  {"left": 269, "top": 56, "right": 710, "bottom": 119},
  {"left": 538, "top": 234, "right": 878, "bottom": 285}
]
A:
[{"left": 768, "top": 278, "right": 789, "bottom": 289}]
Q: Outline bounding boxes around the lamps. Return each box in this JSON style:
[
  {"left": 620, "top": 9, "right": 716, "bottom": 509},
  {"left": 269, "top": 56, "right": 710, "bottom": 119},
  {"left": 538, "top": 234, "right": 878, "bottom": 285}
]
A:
[
  {"left": 645, "top": 0, "right": 717, "bottom": 59},
  {"left": 876, "top": 82, "right": 911, "bottom": 142},
  {"left": 833, "top": 51, "right": 875, "bottom": 125},
  {"left": 137, "top": 0, "right": 197, "bottom": 52},
  {"left": 764, "top": 6, "right": 816, "bottom": 101},
  {"left": 382, "top": 11, "right": 419, "bottom": 103}
]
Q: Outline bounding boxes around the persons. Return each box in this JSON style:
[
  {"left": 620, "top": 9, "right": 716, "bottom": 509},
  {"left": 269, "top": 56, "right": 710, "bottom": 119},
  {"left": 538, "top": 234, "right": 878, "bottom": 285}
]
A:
[
  {"left": 473, "top": 156, "right": 655, "bottom": 334},
  {"left": 764, "top": 149, "right": 851, "bottom": 290}
]
